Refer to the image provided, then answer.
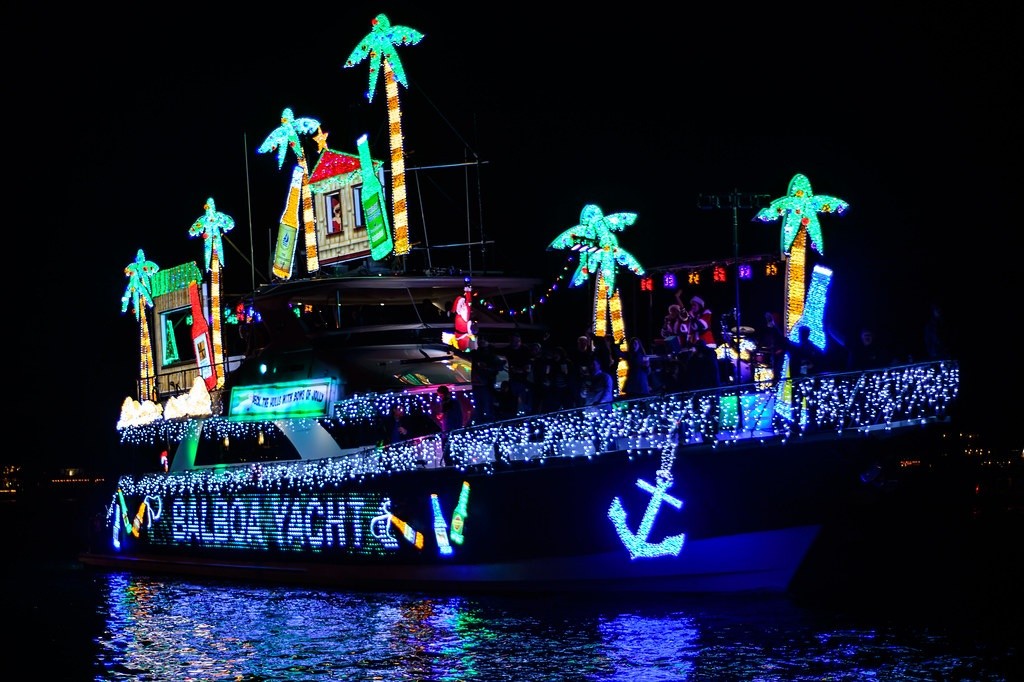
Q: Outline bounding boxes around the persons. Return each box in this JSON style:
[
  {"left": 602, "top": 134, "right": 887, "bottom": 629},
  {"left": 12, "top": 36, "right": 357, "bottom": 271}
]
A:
[{"left": 375, "top": 289, "right": 885, "bottom": 447}]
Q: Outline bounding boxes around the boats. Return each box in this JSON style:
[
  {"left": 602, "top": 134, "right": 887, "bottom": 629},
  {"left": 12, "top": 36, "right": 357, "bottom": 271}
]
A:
[{"left": 104, "top": 132, "right": 961, "bottom": 597}]
